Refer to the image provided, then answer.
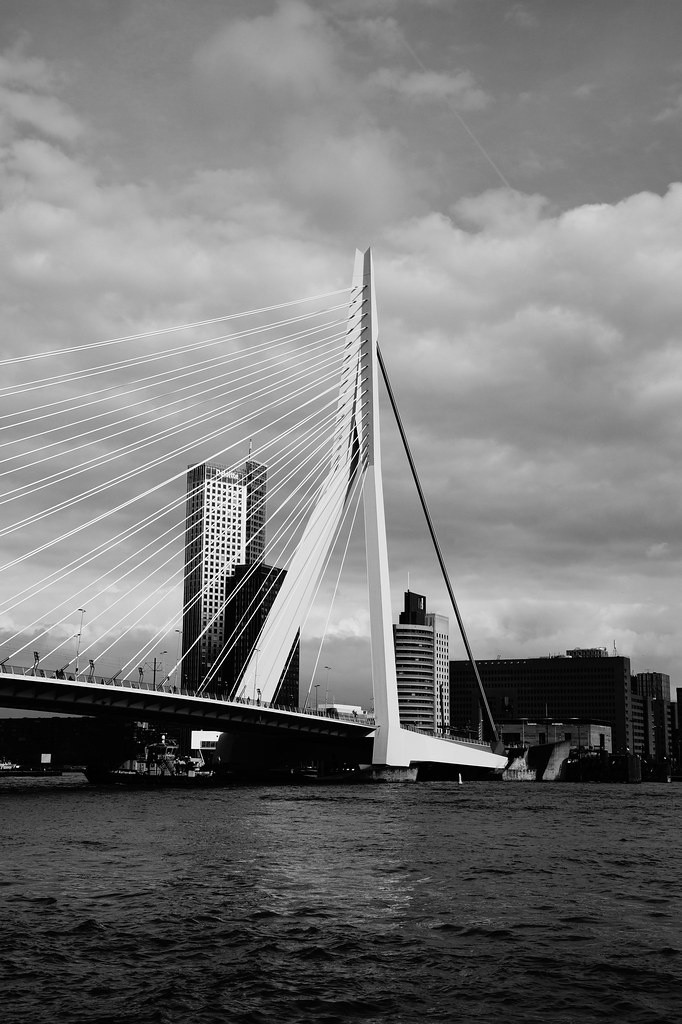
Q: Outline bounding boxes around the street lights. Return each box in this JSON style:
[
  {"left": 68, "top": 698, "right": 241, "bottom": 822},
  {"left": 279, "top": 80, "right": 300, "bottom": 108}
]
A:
[
  {"left": 252, "top": 648, "right": 261, "bottom": 705},
  {"left": 314, "top": 685, "right": 321, "bottom": 715},
  {"left": 324, "top": 666, "right": 332, "bottom": 716},
  {"left": 175, "top": 629, "right": 183, "bottom": 693},
  {"left": 74, "top": 608, "right": 87, "bottom": 681},
  {"left": 159, "top": 651, "right": 168, "bottom": 685}
]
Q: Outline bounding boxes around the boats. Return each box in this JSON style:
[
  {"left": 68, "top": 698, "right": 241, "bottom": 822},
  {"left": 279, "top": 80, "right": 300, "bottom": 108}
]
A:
[
  {"left": 83, "top": 731, "right": 240, "bottom": 788},
  {"left": 263, "top": 761, "right": 375, "bottom": 784},
  {"left": 0, "top": 754, "right": 64, "bottom": 777}
]
